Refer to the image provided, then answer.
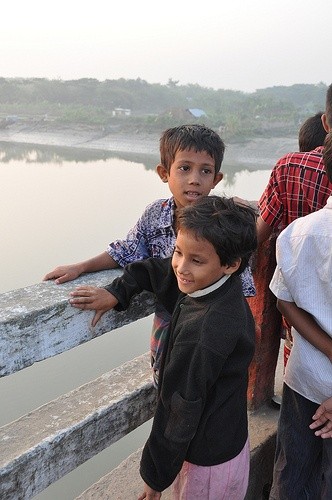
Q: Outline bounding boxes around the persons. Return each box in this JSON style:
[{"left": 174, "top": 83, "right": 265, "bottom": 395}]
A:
[
  {"left": 69, "top": 194, "right": 257, "bottom": 500},
  {"left": 41, "top": 123, "right": 258, "bottom": 392},
  {"left": 254, "top": 83, "right": 332, "bottom": 500}
]
